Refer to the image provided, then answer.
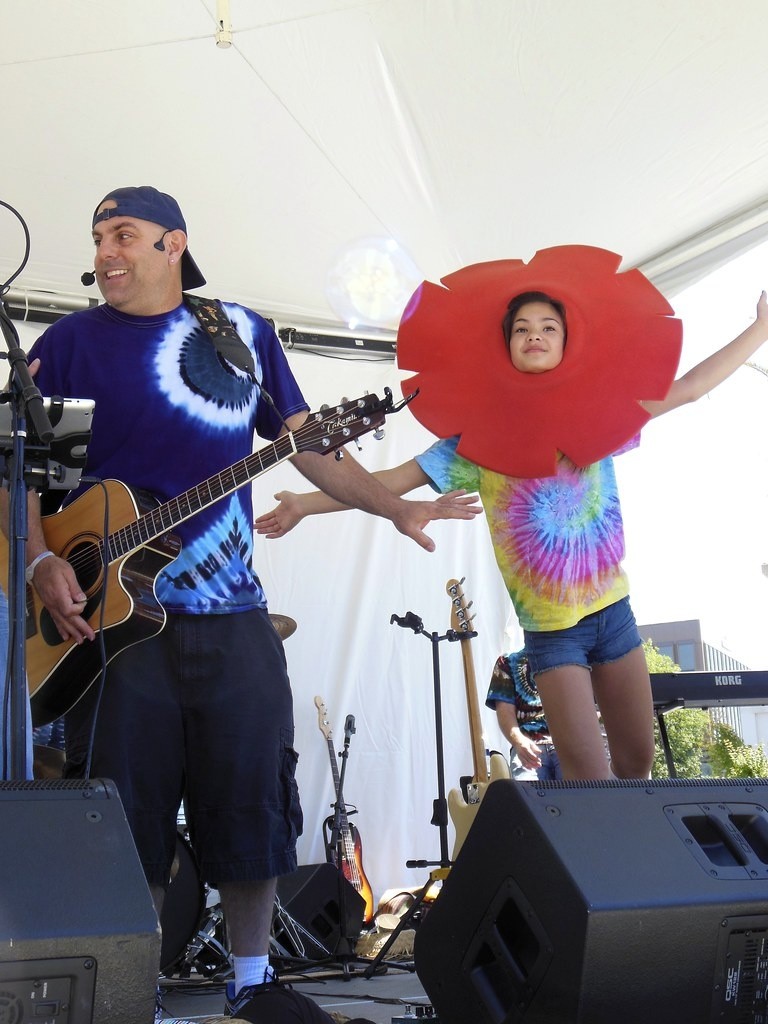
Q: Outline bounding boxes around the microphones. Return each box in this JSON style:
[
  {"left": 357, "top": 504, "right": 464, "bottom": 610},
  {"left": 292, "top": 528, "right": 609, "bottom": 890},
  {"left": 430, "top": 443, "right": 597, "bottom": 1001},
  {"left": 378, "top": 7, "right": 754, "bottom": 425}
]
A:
[
  {"left": 81, "top": 270, "right": 96, "bottom": 286},
  {"left": 342, "top": 714, "right": 355, "bottom": 747}
]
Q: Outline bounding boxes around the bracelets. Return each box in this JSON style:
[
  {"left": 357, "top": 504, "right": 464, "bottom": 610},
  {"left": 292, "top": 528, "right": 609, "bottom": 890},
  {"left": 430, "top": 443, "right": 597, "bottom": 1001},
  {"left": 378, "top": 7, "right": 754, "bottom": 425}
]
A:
[{"left": 25, "top": 552, "right": 55, "bottom": 586}]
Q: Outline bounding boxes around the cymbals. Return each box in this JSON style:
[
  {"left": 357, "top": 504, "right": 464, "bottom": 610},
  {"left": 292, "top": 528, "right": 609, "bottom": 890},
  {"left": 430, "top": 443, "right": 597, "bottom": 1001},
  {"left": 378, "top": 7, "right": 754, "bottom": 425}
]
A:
[{"left": 267, "top": 613, "right": 298, "bottom": 643}]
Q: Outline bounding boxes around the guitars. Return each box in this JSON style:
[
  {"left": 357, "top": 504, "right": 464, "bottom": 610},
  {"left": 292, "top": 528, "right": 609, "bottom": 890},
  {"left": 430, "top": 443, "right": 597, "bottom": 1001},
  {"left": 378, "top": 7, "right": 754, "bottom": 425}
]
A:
[
  {"left": 447, "top": 578, "right": 514, "bottom": 865},
  {"left": 0, "top": 389, "right": 396, "bottom": 731},
  {"left": 312, "top": 692, "right": 377, "bottom": 927}
]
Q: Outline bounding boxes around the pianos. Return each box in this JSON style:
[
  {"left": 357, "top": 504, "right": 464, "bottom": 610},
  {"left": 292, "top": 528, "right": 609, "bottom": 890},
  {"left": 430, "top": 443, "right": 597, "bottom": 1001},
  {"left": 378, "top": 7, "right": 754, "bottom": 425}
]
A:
[{"left": 646, "top": 669, "right": 768, "bottom": 711}]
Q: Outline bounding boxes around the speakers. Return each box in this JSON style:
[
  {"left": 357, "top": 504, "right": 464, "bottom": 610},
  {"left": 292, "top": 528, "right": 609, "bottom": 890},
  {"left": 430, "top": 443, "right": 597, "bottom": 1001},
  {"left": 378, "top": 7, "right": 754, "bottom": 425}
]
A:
[
  {"left": 413, "top": 776, "right": 768, "bottom": 1024},
  {"left": 270, "top": 862, "right": 367, "bottom": 965},
  {"left": 0, "top": 776, "right": 161, "bottom": 1024}
]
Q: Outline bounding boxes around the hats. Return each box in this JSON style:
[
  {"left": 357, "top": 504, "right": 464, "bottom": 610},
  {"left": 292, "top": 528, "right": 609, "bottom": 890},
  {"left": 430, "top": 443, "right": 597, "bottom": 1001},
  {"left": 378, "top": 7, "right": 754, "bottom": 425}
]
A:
[{"left": 91, "top": 186, "right": 207, "bottom": 291}]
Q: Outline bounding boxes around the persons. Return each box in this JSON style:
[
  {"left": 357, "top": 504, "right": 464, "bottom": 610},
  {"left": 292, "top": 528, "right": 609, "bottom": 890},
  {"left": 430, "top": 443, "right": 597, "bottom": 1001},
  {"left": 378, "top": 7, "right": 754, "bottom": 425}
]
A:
[
  {"left": 253, "top": 291, "right": 768, "bottom": 780},
  {"left": 0, "top": 186, "right": 484, "bottom": 1024}
]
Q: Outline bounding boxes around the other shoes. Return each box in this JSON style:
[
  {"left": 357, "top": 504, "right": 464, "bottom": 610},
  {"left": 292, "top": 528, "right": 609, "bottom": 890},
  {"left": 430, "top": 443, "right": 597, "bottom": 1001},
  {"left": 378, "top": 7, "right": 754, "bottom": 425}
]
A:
[
  {"left": 155, "top": 990, "right": 163, "bottom": 1019},
  {"left": 223, "top": 966, "right": 286, "bottom": 1019}
]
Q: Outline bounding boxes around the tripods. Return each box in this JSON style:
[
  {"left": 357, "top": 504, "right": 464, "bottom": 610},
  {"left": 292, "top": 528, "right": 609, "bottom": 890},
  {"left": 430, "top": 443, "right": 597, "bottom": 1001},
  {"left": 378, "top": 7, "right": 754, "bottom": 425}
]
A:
[{"left": 177, "top": 611, "right": 478, "bottom": 982}]
